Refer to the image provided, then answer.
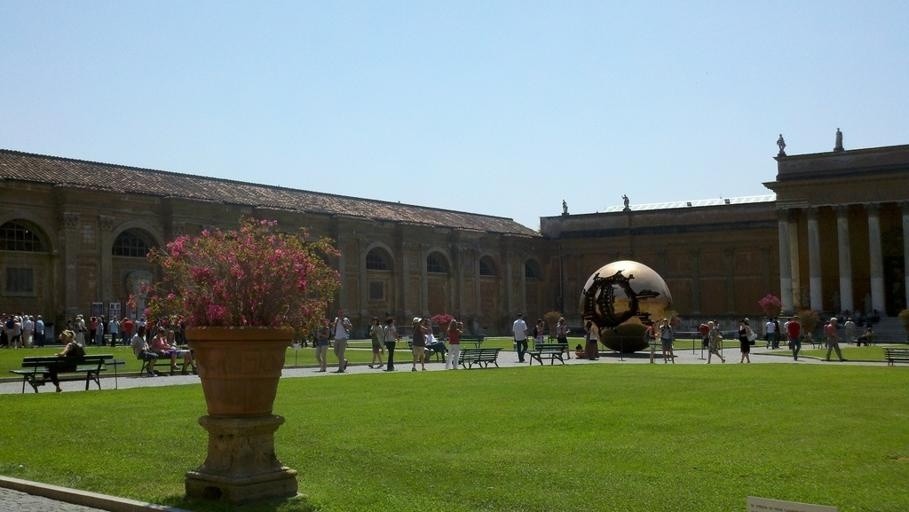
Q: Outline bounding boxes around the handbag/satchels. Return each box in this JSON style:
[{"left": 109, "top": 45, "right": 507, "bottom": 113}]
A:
[{"left": 745, "top": 327, "right": 755, "bottom": 342}]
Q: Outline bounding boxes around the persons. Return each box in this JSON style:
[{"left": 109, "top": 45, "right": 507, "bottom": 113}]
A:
[
  {"left": 507, "top": 312, "right": 601, "bottom": 362},
  {"left": 1, "top": 310, "right": 200, "bottom": 392},
  {"left": 761, "top": 293, "right": 881, "bottom": 361},
  {"left": 642, "top": 306, "right": 756, "bottom": 365},
  {"left": 301, "top": 309, "right": 351, "bottom": 374},
  {"left": 369, "top": 315, "right": 466, "bottom": 370}
]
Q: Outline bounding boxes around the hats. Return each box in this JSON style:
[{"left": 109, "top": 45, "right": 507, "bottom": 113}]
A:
[
  {"left": 62, "top": 330, "right": 75, "bottom": 342},
  {"left": 413, "top": 317, "right": 422, "bottom": 323}
]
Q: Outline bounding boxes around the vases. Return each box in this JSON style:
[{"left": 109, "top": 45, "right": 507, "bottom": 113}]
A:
[{"left": 173, "top": 318, "right": 297, "bottom": 421}]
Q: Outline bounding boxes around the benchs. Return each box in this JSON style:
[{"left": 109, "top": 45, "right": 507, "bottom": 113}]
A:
[
  {"left": 524, "top": 342, "right": 569, "bottom": 366},
  {"left": 809, "top": 336, "right": 822, "bottom": 349},
  {"left": 453, "top": 348, "right": 503, "bottom": 369},
  {"left": 883, "top": 345, "right": 909, "bottom": 367},
  {"left": 136, "top": 351, "right": 199, "bottom": 375},
  {"left": 407, "top": 340, "right": 441, "bottom": 362},
  {"left": 11, "top": 351, "right": 126, "bottom": 392},
  {"left": 862, "top": 331, "right": 874, "bottom": 347},
  {"left": 458, "top": 335, "right": 485, "bottom": 349}
]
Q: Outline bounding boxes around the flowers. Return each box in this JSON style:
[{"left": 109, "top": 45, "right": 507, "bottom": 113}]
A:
[
  {"left": 758, "top": 293, "right": 783, "bottom": 320},
  {"left": 115, "top": 213, "right": 344, "bottom": 345}
]
